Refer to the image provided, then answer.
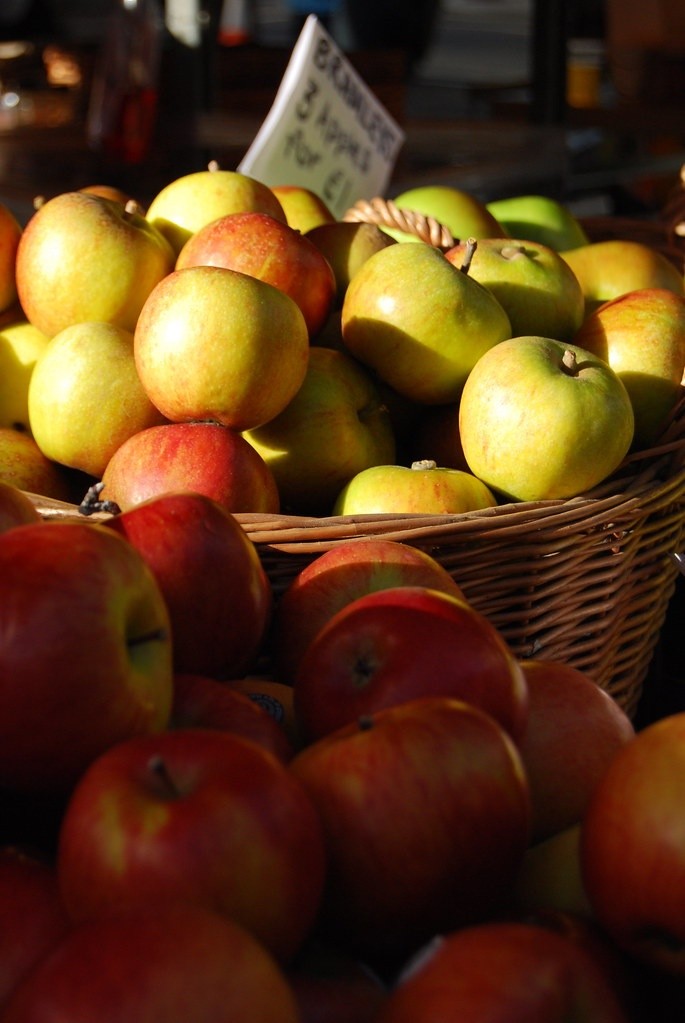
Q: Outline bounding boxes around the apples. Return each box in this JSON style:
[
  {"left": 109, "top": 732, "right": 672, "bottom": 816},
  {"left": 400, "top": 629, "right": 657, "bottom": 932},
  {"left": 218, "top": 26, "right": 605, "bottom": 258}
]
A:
[
  {"left": 0, "top": 489, "right": 685, "bottom": 1023},
  {"left": 1, "top": 159, "right": 685, "bottom": 516}
]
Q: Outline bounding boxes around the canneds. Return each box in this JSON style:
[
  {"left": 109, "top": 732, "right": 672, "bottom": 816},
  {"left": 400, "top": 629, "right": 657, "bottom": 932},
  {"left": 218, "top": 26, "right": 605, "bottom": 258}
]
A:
[{"left": 562, "top": 37, "right": 604, "bottom": 107}]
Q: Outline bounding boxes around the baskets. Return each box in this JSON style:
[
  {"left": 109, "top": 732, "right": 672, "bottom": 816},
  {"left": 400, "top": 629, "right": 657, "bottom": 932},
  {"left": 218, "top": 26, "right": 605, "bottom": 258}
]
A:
[{"left": 3, "top": 197, "right": 685, "bottom": 722}]
